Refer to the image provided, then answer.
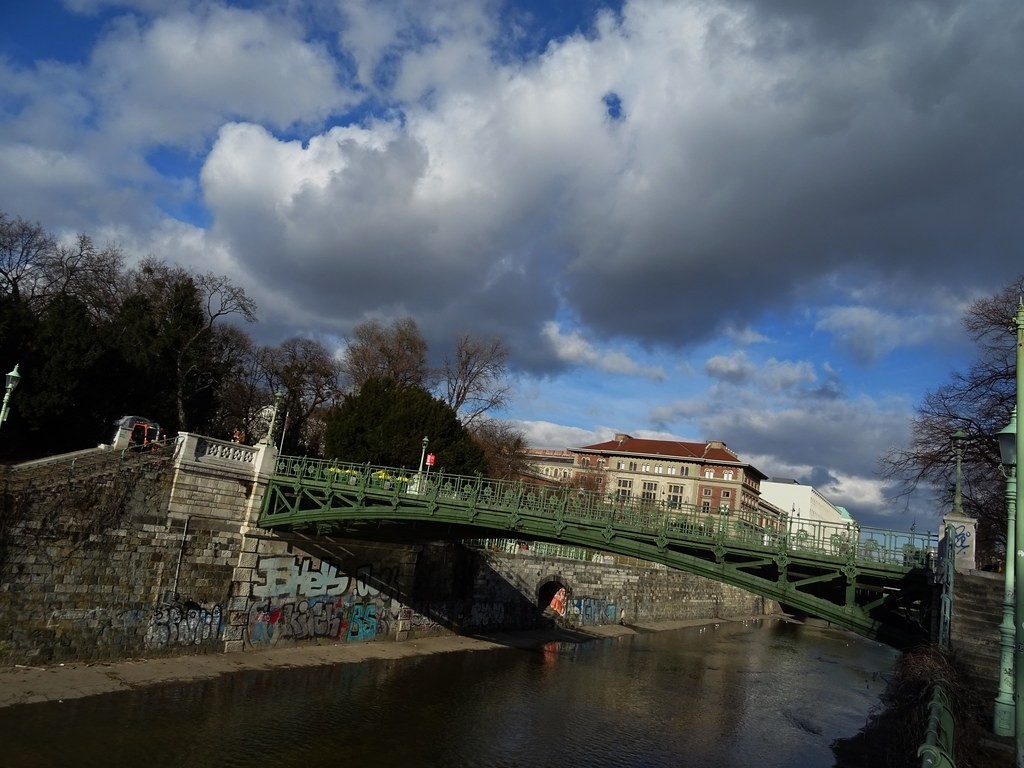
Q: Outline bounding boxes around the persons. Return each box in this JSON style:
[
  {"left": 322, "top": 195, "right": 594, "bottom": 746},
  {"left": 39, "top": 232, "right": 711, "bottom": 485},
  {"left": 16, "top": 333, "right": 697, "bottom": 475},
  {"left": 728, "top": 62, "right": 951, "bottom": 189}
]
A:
[
  {"left": 620, "top": 609, "right": 626, "bottom": 625},
  {"left": 515, "top": 543, "right": 519, "bottom": 554},
  {"left": 521, "top": 543, "right": 527, "bottom": 555}
]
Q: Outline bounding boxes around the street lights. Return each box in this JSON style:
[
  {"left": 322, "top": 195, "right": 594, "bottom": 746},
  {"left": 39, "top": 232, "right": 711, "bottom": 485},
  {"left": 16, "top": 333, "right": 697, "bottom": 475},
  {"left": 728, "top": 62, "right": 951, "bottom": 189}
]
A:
[
  {"left": 995, "top": 410, "right": 1019, "bottom": 742},
  {"left": 685, "top": 496, "right": 689, "bottom": 529},
  {"left": 1, "top": 363, "right": 20, "bottom": 428},
  {"left": 412, "top": 436, "right": 430, "bottom": 480},
  {"left": 657, "top": 485, "right": 665, "bottom": 526},
  {"left": 796, "top": 507, "right": 799, "bottom": 544},
  {"left": 259, "top": 389, "right": 285, "bottom": 447},
  {"left": 789, "top": 501, "right": 796, "bottom": 545},
  {"left": 911, "top": 520, "right": 917, "bottom": 545},
  {"left": 948, "top": 427, "right": 968, "bottom": 517}
]
